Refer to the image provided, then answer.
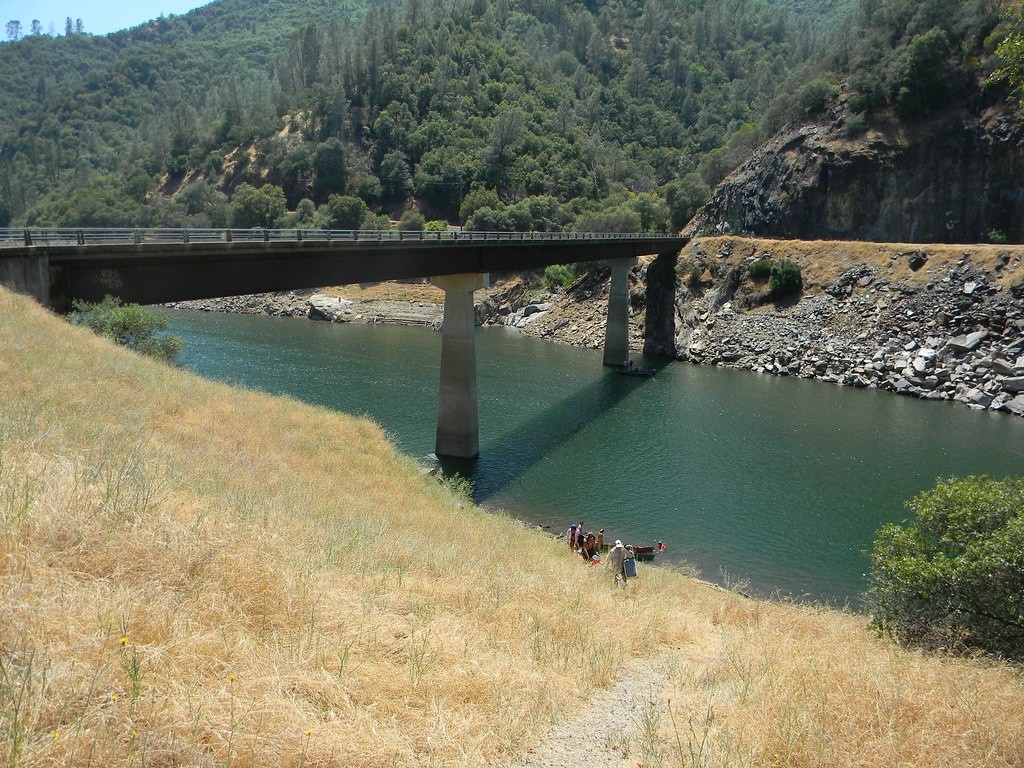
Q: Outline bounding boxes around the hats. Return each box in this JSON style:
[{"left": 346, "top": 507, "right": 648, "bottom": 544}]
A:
[
  {"left": 614, "top": 540, "right": 623, "bottom": 548},
  {"left": 569, "top": 524, "right": 576, "bottom": 531}
]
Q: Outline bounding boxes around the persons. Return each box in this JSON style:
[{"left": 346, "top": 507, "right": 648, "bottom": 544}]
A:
[
  {"left": 578, "top": 521, "right": 584, "bottom": 535},
  {"left": 567, "top": 524, "right": 581, "bottom": 549},
  {"left": 583, "top": 532, "right": 600, "bottom": 561},
  {"left": 605, "top": 539, "right": 630, "bottom": 590},
  {"left": 657, "top": 541, "right": 662, "bottom": 549},
  {"left": 597, "top": 529, "right": 604, "bottom": 549}
]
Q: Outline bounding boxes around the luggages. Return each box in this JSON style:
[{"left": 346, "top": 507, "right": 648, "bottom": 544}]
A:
[{"left": 624, "top": 557, "right": 636, "bottom": 577}]
